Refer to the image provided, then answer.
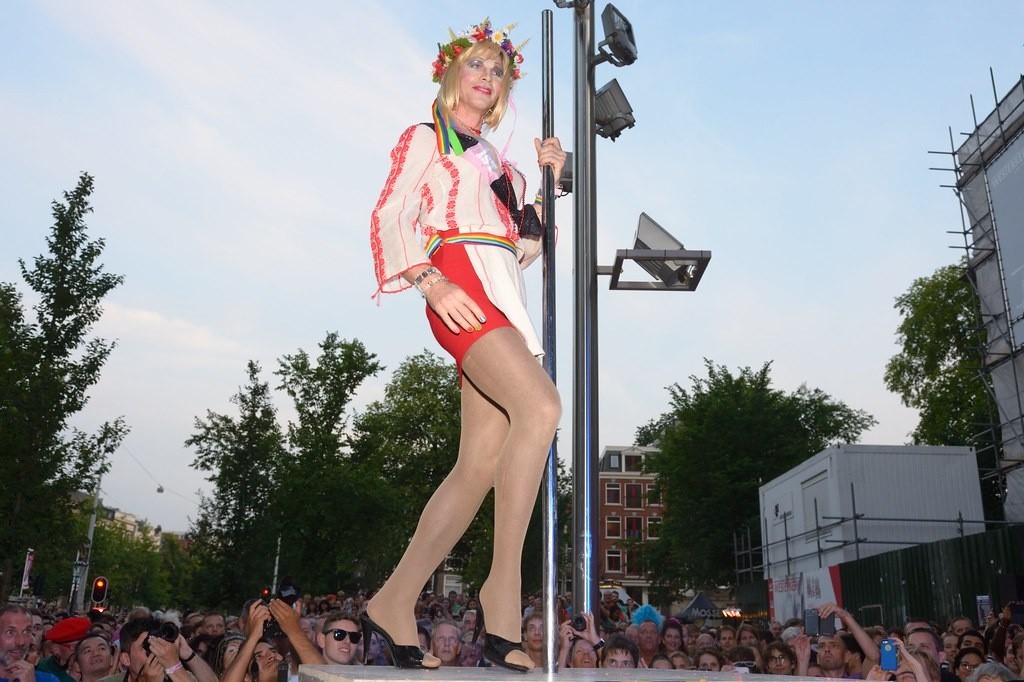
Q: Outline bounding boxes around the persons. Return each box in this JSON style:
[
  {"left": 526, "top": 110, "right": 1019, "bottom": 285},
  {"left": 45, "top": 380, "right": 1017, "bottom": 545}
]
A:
[
  {"left": 0, "top": 587, "right": 1024, "bottom": 682},
  {"left": 359, "top": 16, "right": 566, "bottom": 672}
]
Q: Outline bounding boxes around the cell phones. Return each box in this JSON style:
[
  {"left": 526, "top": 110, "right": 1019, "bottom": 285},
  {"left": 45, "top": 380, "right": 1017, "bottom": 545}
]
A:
[
  {"left": 1010, "top": 603, "right": 1024, "bottom": 614},
  {"left": 729, "top": 667, "right": 749, "bottom": 674},
  {"left": 804, "top": 609, "right": 820, "bottom": 637},
  {"left": 880, "top": 639, "right": 897, "bottom": 670}
]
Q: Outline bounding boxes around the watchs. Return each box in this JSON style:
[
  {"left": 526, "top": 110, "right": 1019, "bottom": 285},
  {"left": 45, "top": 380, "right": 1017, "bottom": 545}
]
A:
[
  {"left": 593, "top": 639, "right": 605, "bottom": 649},
  {"left": 540, "top": 180, "right": 563, "bottom": 198}
]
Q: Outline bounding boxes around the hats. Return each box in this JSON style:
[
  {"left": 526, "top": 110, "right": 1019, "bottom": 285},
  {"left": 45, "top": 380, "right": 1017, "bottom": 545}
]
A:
[
  {"left": 326, "top": 594, "right": 337, "bottom": 599},
  {"left": 44, "top": 616, "right": 91, "bottom": 644}
]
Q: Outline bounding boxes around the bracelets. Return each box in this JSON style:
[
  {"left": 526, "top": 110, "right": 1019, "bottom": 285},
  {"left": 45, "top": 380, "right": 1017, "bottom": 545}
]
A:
[
  {"left": 534, "top": 193, "right": 542, "bottom": 206},
  {"left": 413, "top": 266, "right": 450, "bottom": 298},
  {"left": 164, "top": 662, "right": 182, "bottom": 673},
  {"left": 183, "top": 652, "right": 195, "bottom": 663}
]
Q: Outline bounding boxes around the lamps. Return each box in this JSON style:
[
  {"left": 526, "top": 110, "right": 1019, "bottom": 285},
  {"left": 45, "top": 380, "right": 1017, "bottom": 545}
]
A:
[
  {"left": 595, "top": 3, "right": 638, "bottom": 68},
  {"left": 557, "top": 152, "right": 573, "bottom": 193},
  {"left": 594, "top": 78, "right": 637, "bottom": 142},
  {"left": 631, "top": 212, "right": 690, "bottom": 288}
]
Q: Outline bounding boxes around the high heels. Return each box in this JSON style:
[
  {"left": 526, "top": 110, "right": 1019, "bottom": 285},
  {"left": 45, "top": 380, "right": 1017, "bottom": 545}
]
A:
[
  {"left": 358, "top": 612, "right": 440, "bottom": 670},
  {"left": 471, "top": 589, "right": 530, "bottom": 672}
]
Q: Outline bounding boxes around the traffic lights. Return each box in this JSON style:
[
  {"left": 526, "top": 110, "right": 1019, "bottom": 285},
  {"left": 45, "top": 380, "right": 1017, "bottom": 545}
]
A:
[
  {"left": 261, "top": 586, "right": 272, "bottom": 602},
  {"left": 91, "top": 577, "right": 108, "bottom": 603}
]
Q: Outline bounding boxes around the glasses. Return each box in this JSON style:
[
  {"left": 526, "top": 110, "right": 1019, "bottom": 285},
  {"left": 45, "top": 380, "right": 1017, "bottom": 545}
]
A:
[
  {"left": 732, "top": 661, "right": 757, "bottom": 668},
  {"left": 767, "top": 654, "right": 789, "bottom": 664},
  {"left": 960, "top": 661, "right": 979, "bottom": 672},
  {"left": 324, "top": 628, "right": 363, "bottom": 644}
]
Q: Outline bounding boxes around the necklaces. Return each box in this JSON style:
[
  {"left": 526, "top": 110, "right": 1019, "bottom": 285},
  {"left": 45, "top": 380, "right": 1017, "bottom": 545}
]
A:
[{"left": 469, "top": 126, "right": 526, "bottom": 234}]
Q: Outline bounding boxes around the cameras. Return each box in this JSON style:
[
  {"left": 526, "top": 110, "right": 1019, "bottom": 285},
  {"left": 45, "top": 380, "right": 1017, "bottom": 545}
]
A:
[
  {"left": 143, "top": 621, "right": 179, "bottom": 656},
  {"left": 244, "top": 583, "right": 300, "bottom": 638},
  {"left": 567, "top": 616, "right": 587, "bottom": 641}
]
